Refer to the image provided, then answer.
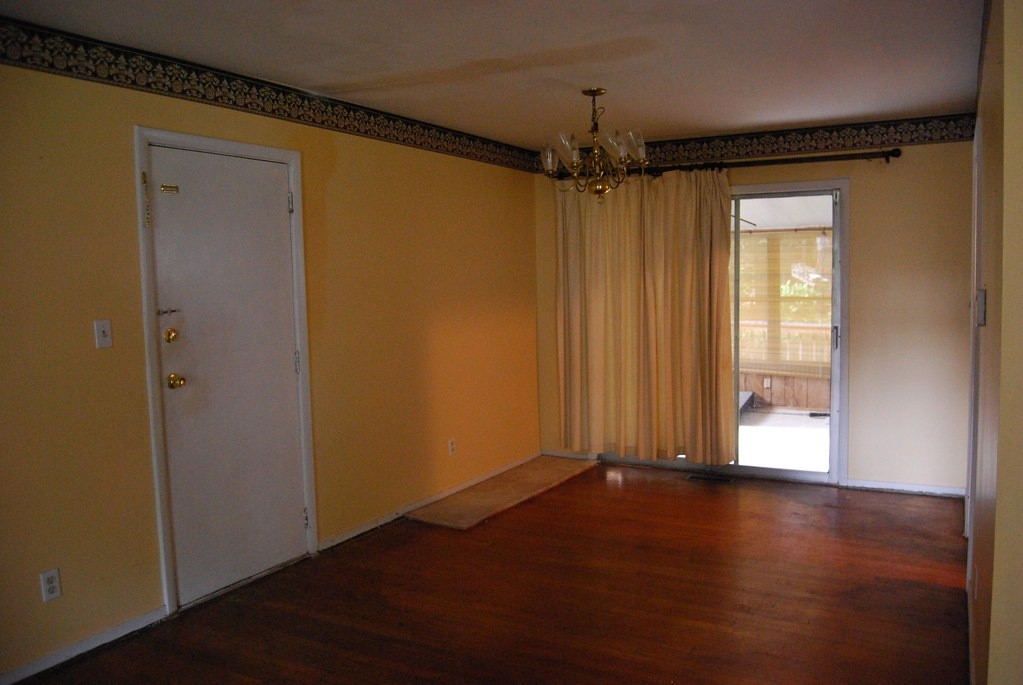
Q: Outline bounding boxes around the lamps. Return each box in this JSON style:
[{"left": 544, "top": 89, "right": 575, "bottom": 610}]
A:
[{"left": 540, "top": 87, "right": 647, "bottom": 205}]
